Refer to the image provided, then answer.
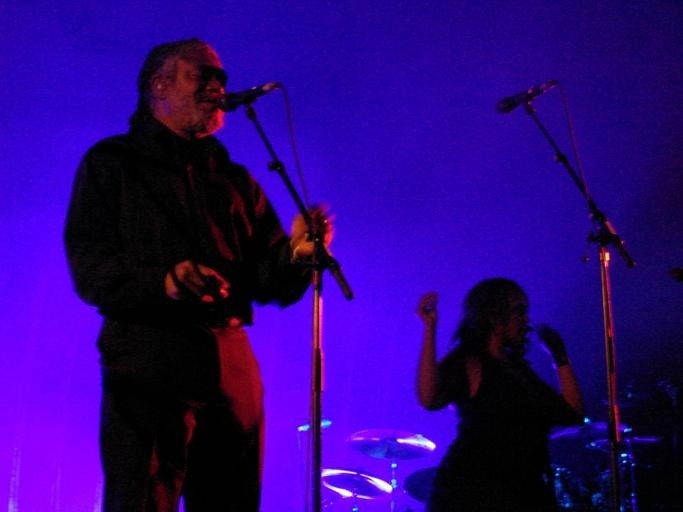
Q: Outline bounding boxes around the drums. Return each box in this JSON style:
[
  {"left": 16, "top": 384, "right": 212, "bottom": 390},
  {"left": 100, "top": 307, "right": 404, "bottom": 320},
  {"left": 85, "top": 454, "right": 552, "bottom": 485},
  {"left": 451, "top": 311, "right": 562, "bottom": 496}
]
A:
[{"left": 556, "top": 467, "right": 633, "bottom": 512}]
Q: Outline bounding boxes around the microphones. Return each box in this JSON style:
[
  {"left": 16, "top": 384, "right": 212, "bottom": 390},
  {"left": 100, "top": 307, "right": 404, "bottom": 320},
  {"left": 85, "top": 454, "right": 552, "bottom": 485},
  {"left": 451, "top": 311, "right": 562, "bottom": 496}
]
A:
[
  {"left": 496, "top": 78, "right": 558, "bottom": 113},
  {"left": 216, "top": 79, "right": 278, "bottom": 113}
]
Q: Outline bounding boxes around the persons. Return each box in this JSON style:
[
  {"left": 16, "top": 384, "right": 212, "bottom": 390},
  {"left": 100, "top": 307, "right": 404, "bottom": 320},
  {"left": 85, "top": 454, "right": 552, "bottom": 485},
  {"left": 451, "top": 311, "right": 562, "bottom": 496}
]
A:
[
  {"left": 64, "top": 39, "right": 335, "bottom": 512},
  {"left": 414, "top": 276, "right": 585, "bottom": 512}
]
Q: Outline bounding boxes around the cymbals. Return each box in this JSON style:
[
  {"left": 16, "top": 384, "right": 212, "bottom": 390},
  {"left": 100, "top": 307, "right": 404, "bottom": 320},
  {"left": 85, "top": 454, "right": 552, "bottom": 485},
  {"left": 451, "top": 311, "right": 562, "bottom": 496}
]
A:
[
  {"left": 322, "top": 468, "right": 394, "bottom": 501},
  {"left": 548, "top": 422, "right": 632, "bottom": 449},
  {"left": 583, "top": 436, "right": 662, "bottom": 454},
  {"left": 344, "top": 428, "right": 436, "bottom": 459},
  {"left": 403, "top": 467, "right": 441, "bottom": 501}
]
